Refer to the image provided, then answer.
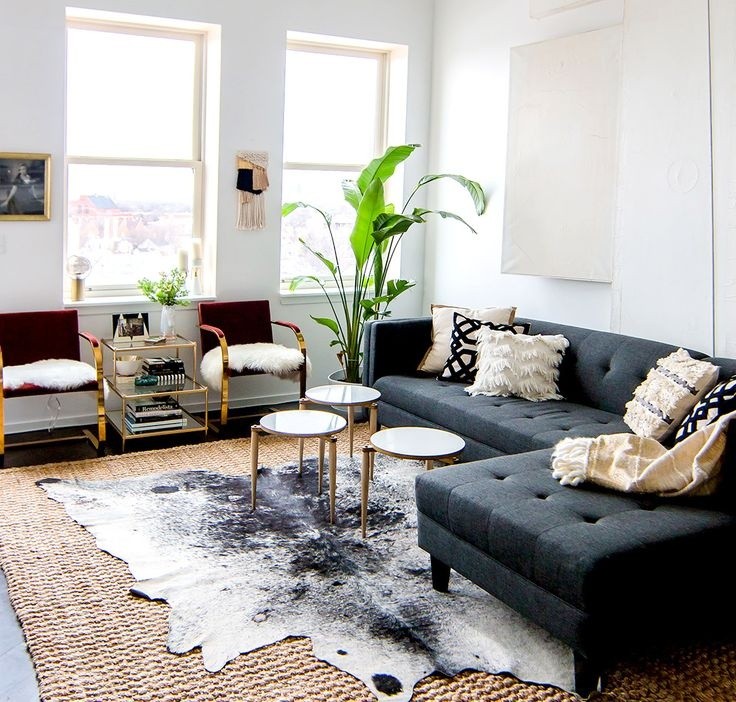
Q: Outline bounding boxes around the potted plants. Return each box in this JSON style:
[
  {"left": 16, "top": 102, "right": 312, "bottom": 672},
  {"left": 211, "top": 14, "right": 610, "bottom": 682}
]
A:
[
  {"left": 138, "top": 267, "right": 191, "bottom": 340},
  {"left": 282, "top": 143, "right": 488, "bottom": 413}
]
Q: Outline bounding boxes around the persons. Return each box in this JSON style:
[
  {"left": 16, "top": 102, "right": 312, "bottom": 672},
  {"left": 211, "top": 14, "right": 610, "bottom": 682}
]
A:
[{"left": 0, "top": 163, "right": 43, "bottom": 215}]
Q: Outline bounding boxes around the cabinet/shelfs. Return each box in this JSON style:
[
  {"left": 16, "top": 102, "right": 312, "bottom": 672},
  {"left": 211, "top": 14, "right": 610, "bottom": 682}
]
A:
[{"left": 101, "top": 334, "right": 208, "bottom": 451}]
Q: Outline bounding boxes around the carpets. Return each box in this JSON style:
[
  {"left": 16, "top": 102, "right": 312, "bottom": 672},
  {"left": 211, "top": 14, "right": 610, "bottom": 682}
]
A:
[{"left": 1, "top": 421, "right": 736, "bottom": 702}]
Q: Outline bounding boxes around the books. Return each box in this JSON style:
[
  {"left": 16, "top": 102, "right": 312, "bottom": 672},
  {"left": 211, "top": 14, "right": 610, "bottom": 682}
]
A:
[
  {"left": 142, "top": 357, "right": 186, "bottom": 380},
  {"left": 144, "top": 336, "right": 166, "bottom": 343},
  {"left": 124, "top": 396, "right": 188, "bottom": 433}
]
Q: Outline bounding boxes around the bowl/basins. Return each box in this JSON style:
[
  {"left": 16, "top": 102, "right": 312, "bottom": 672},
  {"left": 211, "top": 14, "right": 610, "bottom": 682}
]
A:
[{"left": 113, "top": 355, "right": 143, "bottom": 376}]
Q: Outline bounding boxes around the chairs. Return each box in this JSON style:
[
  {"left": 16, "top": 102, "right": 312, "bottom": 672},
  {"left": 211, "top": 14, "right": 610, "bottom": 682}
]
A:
[
  {"left": 0, "top": 309, "right": 106, "bottom": 453},
  {"left": 198, "top": 299, "right": 307, "bottom": 423}
]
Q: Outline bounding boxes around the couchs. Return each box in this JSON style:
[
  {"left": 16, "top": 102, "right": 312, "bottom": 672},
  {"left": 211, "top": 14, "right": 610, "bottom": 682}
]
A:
[{"left": 364, "top": 308, "right": 736, "bottom": 697}]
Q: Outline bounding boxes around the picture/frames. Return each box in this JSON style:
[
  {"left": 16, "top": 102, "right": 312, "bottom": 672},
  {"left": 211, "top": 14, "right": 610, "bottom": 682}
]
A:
[{"left": 0, "top": 153, "right": 52, "bottom": 220}]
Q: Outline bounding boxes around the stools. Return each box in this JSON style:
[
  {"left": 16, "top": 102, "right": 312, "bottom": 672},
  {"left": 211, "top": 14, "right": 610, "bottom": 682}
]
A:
[
  {"left": 250, "top": 410, "right": 347, "bottom": 525},
  {"left": 296, "top": 384, "right": 382, "bottom": 492},
  {"left": 362, "top": 427, "right": 466, "bottom": 538}
]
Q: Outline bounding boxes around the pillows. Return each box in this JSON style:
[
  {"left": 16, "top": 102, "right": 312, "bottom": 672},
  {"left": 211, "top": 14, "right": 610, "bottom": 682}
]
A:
[
  {"left": 464, "top": 325, "right": 570, "bottom": 401},
  {"left": 622, "top": 348, "right": 723, "bottom": 443},
  {"left": 675, "top": 375, "right": 736, "bottom": 441},
  {"left": 435, "top": 312, "right": 531, "bottom": 383},
  {"left": 416, "top": 305, "right": 516, "bottom": 375}
]
